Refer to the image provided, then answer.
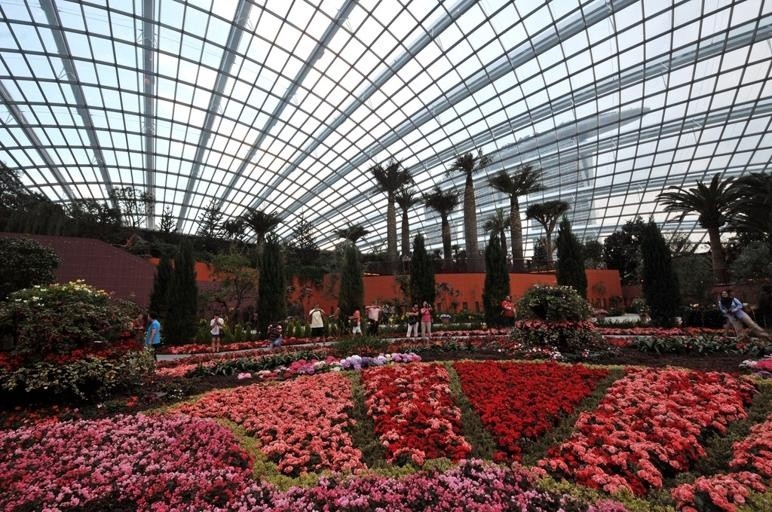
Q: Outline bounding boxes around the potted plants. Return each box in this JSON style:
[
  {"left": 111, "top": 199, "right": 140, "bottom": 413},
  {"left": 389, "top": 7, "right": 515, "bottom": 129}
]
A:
[
  {"left": 439, "top": 314, "right": 451, "bottom": 324},
  {"left": 637, "top": 303, "right": 651, "bottom": 321},
  {"left": 594, "top": 309, "right": 608, "bottom": 322}
]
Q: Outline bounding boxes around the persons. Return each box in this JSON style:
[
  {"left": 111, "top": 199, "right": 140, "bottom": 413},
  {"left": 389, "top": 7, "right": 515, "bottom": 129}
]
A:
[
  {"left": 146, "top": 311, "right": 163, "bottom": 360},
  {"left": 501, "top": 295, "right": 517, "bottom": 326},
  {"left": 209, "top": 314, "right": 225, "bottom": 353},
  {"left": 365, "top": 300, "right": 383, "bottom": 334},
  {"left": 718, "top": 290, "right": 770, "bottom": 338},
  {"left": 405, "top": 302, "right": 420, "bottom": 338},
  {"left": 419, "top": 301, "right": 434, "bottom": 337},
  {"left": 350, "top": 309, "right": 364, "bottom": 337},
  {"left": 309, "top": 302, "right": 326, "bottom": 344}
]
